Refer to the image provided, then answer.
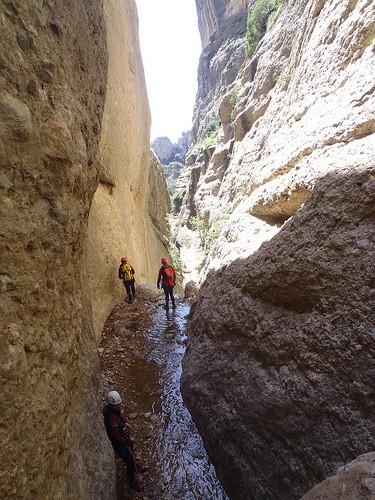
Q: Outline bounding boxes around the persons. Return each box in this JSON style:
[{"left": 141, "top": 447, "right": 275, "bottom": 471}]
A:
[
  {"left": 157, "top": 257, "right": 176, "bottom": 310},
  {"left": 103, "top": 390, "right": 148, "bottom": 492},
  {"left": 118, "top": 258, "right": 136, "bottom": 304}
]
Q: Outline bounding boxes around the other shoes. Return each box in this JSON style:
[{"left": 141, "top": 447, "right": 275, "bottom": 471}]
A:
[
  {"left": 138, "top": 465, "right": 149, "bottom": 473},
  {"left": 130, "top": 481, "right": 145, "bottom": 492}
]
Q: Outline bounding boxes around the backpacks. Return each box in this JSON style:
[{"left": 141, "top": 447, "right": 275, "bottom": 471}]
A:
[
  {"left": 121, "top": 264, "right": 133, "bottom": 281},
  {"left": 161, "top": 266, "right": 174, "bottom": 287}
]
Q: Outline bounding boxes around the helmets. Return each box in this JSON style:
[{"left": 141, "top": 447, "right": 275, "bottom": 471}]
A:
[
  {"left": 161, "top": 257, "right": 167, "bottom": 264},
  {"left": 107, "top": 391, "right": 122, "bottom": 404},
  {"left": 121, "top": 257, "right": 127, "bottom": 261}
]
